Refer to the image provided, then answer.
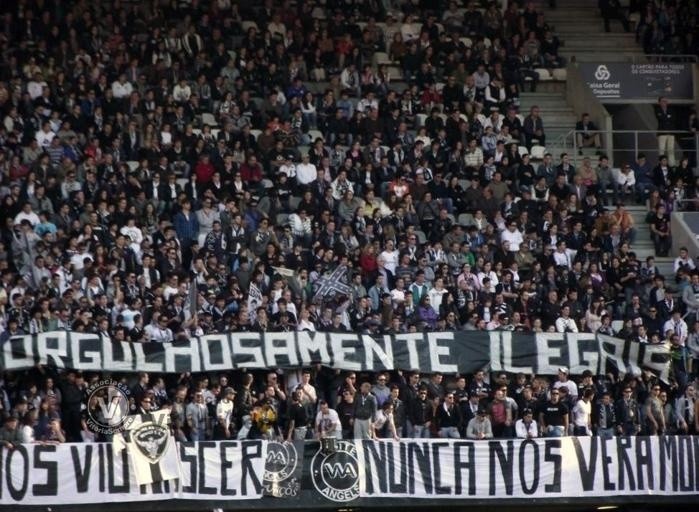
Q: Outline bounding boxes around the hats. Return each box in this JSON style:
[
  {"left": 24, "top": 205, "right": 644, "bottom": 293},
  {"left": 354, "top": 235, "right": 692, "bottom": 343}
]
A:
[
  {"left": 559, "top": 367, "right": 570, "bottom": 375},
  {"left": 225, "top": 387, "right": 237, "bottom": 394}
]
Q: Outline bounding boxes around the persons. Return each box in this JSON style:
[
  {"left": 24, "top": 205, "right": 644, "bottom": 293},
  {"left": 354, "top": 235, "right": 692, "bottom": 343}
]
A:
[{"left": 1, "top": 1, "right": 699, "bottom": 451}]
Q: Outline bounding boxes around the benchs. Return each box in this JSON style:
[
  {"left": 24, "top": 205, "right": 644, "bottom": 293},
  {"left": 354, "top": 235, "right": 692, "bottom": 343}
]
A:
[
  {"left": 518, "top": 91, "right": 580, "bottom": 152},
  {"left": 540, "top": 1, "right": 646, "bottom": 64},
  {"left": 599, "top": 202, "right": 677, "bottom": 280}
]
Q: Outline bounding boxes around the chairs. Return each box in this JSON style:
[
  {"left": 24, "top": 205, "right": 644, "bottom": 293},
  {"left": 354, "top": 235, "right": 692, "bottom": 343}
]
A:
[{"left": 533, "top": 66, "right": 568, "bottom": 83}]
